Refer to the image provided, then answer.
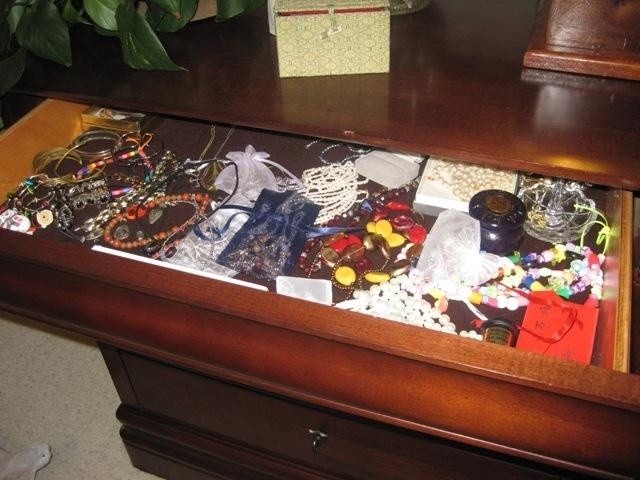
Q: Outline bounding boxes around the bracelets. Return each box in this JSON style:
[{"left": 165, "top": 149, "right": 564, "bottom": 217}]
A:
[{"left": 1, "top": 123, "right": 163, "bottom": 234}]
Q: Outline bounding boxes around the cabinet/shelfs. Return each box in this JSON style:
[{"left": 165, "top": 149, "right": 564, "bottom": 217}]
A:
[{"left": 1, "top": 0, "right": 640, "bottom": 479}]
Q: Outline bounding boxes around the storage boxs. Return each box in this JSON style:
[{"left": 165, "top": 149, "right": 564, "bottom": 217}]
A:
[{"left": 274, "top": 0, "right": 393, "bottom": 80}]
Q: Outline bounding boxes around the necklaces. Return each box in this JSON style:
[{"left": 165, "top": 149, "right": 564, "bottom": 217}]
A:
[{"left": 72, "top": 122, "right": 610, "bottom": 345}]
